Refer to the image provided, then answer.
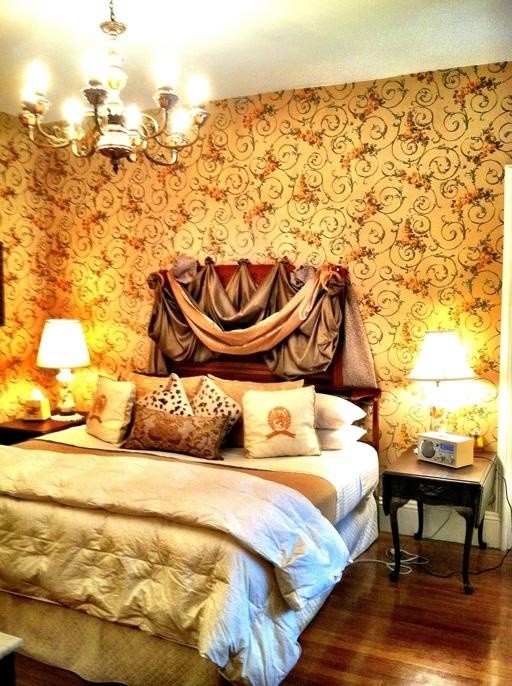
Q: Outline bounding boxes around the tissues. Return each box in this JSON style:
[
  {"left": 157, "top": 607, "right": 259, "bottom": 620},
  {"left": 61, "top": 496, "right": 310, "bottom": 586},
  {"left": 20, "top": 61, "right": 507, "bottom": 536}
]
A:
[{"left": 23, "top": 387, "right": 51, "bottom": 420}]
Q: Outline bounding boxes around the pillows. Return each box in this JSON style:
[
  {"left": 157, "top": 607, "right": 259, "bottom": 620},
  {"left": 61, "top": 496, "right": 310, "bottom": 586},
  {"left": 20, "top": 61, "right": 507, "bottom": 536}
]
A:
[
  {"left": 314, "top": 392, "right": 367, "bottom": 431},
  {"left": 119, "top": 370, "right": 204, "bottom": 401},
  {"left": 118, "top": 402, "right": 230, "bottom": 462},
  {"left": 314, "top": 424, "right": 369, "bottom": 450},
  {"left": 241, "top": 384, "right": 322, "bottom": 460},
  {"left": 136, "top": 373, "right": 196, "bottom": 417},
  {"left": 189, "top": 376, "right": 242, "bottom": 444},
  {"left": 85, "top": 374, "right": 137, "bottom": 444},
  {"left": 207, "top": 373, "right": 304, "bottom": 449}
]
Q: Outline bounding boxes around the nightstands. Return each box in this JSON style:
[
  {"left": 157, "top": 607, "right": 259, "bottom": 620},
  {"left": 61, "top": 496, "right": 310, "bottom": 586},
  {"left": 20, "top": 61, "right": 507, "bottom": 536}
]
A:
[
  {"left": 0, "top": 410, "right": 90, "bottom": 446},
  {"left": 381, "top": 443, "right": 498, "bottom": 596}
]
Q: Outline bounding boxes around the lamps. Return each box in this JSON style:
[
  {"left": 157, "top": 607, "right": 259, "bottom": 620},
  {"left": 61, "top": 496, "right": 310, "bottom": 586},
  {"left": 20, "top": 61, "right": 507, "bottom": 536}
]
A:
[
  {"left": 18, "top": 23, "right": 216, "bottom": 173},
  {"left": 35, "top": 319, "right": 92, "bottom": 424},
  {"left": 403, "top": 330, "right": 479, "bottom": 431}
]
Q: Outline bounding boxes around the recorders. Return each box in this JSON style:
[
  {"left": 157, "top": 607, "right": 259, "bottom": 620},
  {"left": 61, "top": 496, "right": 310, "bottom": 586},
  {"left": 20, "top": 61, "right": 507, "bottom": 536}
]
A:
[{"left": 416, "top": 432, "right": 473, "bottom": 468}]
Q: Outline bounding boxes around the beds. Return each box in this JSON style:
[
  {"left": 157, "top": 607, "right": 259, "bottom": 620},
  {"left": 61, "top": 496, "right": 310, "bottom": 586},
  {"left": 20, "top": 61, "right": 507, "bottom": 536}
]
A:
[{"left": 1, "top": 256, "right": 380, "bottom": 686}]
